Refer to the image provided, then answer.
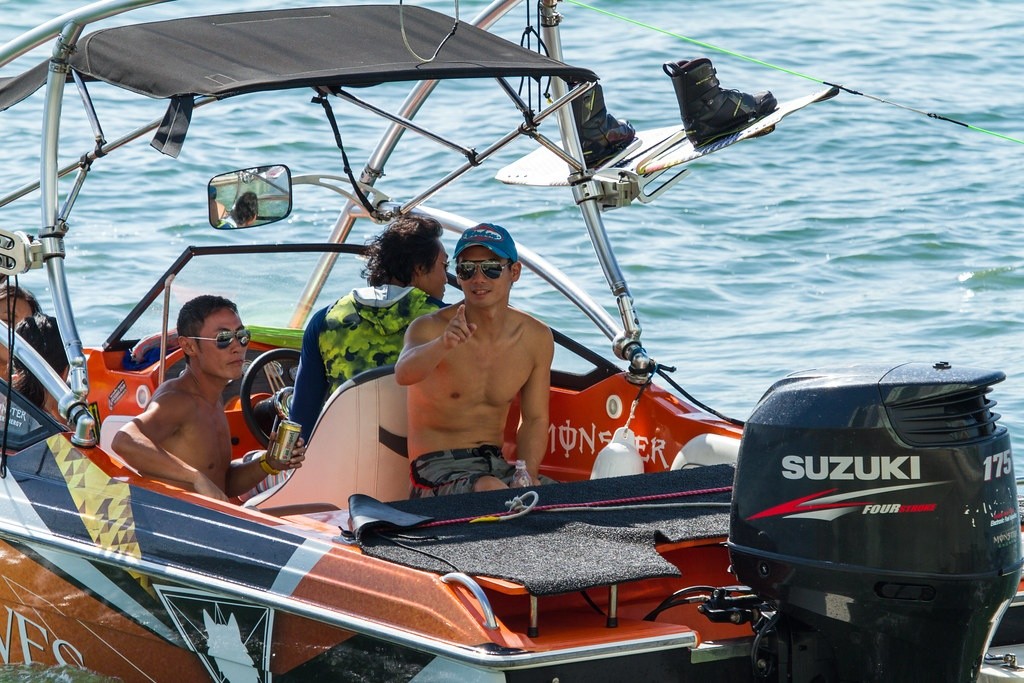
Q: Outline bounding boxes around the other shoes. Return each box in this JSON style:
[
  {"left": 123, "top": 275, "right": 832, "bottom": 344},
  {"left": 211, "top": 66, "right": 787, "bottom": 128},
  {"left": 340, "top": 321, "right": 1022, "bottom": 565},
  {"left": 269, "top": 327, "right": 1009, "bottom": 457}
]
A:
[
  {"left": 663, "top": 58, "right": 777, "bottom": 150},
  {"left": 568, "top": 84, "right": 635, "bottom": 171}
]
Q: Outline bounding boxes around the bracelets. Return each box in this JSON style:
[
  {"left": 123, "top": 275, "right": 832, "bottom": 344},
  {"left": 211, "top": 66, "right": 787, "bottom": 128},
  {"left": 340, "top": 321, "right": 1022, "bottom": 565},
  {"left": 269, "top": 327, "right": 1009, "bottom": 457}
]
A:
[{"left": 260, "top": 453, "right": 280, "bottom": 476}]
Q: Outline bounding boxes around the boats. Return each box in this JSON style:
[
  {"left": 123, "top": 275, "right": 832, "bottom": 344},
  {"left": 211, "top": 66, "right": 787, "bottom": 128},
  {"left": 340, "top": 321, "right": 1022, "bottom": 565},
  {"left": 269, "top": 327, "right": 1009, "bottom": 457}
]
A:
[{"left": 0, "top": 0, "right": 1024, "bottom": 683}]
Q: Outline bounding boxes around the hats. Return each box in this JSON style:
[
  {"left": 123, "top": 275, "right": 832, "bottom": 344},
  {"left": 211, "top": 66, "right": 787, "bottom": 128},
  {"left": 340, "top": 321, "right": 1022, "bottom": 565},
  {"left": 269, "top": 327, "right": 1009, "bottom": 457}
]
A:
[{"left": 453, "top": 223, "right": 517, "bottom": 263}]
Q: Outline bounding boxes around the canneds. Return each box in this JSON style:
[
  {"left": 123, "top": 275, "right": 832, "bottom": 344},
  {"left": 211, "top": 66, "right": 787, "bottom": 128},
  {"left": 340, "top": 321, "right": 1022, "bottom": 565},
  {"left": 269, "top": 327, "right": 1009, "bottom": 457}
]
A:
[{"left": 270, "top": 420, "right": 302, "bottom": 466}]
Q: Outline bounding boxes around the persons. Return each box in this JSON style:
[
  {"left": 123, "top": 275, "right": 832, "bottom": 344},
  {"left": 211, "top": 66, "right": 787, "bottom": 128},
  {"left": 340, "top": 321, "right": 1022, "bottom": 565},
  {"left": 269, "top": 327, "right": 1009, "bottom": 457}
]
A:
[
  {"left": 0, "top": 313, "right": 73, "bottom": 455},
  {"left": 111, "top": 295, "right": 307, "bottom": 502},
  {"left": 0, "top": 284, "right": 43, "bottom": 385},
  {"left": 210, "top": 186, "right": 259, "bottom": 228},
  {"left": 394, "top": 222, "right": 564, "bottom": 498},
  {"left": 228, "top": 216, "right": 453, "bottom": 503}
]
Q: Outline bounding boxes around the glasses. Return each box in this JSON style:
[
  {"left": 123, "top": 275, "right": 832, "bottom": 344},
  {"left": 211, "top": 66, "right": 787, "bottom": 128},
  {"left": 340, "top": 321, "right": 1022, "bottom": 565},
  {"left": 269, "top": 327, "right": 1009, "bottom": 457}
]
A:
[
  {"left": 187, "top": 328, "right": 251, "bottom": 350},
  {"left": 456, "top": 259, "right": 513, "bottom": 281}
]
